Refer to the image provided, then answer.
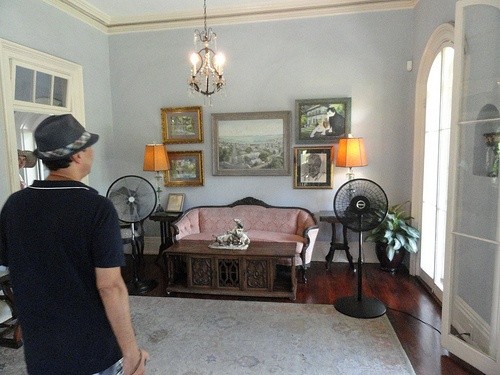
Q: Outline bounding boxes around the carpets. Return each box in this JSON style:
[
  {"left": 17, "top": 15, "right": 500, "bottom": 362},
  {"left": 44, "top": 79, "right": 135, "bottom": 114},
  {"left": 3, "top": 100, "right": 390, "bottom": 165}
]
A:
[{"left": 0, "top": 295, "right": 419, "bottom": 375}]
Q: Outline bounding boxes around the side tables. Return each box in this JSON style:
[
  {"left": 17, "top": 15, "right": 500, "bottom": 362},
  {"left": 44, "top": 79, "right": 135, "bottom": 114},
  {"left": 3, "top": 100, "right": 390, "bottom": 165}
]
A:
[
  {"left": 147, "top": 210, "right": 182, "bottom": 261},
  {"left": 317, "top": 210, "right": 359, "bottom": 274}
]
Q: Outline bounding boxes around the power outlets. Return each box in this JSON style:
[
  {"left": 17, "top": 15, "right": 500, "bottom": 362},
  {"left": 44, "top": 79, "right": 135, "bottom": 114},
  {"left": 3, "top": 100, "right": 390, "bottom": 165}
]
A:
[{"left": 467, "top": 329, "right": 473, "bottom": 341}]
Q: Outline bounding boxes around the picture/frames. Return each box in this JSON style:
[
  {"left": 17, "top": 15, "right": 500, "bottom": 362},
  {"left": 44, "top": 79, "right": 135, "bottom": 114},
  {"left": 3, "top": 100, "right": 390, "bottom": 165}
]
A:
[
  {"left": 208, "top": 109, "right": 296, "bottom": 178},
  {"left": 165, "top": 193, "right": 186, "bottom": 212},
  {"left": 293, "top": 96, "right": 353, "bottom": 146},
  {"left": 292, "top": 144, "right": 335, "bottom": 190},
  {"left": 160, "top": 106, "right": 204, "bottom": 145},
  {"left": 163, "top": 149, "right": 205, "bottom": 188}
]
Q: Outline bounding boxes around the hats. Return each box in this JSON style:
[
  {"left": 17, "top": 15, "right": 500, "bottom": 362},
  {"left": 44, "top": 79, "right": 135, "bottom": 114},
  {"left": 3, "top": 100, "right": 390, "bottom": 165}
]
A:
[{"left": 33, "top": 114, "right": 99, "bottom": 160}]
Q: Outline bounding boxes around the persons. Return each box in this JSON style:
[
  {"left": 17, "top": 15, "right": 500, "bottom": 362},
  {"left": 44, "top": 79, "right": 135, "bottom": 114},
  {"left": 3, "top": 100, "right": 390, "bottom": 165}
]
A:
[
  {"left": 0, "top": 113, "right": 150, "bottom": 375},
  {"left": 17, "top": 148, "right": 38, "bottom": 190}
]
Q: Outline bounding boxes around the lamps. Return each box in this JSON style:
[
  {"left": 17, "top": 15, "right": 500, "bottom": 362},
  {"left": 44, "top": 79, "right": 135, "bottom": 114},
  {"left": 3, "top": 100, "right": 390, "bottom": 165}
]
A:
[
  {"left": 336, "top": 136, "right": 370, "bottom": 212},
  {"left": 143, "top": 142, "right": 170, "bottom": 214},
  {"left": 184, "top": 0, "right": 227, "bottom": 98}
]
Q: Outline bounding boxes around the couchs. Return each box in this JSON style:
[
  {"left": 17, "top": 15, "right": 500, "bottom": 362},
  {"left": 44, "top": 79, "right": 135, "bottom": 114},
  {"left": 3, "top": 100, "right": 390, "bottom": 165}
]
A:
[{"left": 169, "top": 196, "right": 321, "bottom": 284}]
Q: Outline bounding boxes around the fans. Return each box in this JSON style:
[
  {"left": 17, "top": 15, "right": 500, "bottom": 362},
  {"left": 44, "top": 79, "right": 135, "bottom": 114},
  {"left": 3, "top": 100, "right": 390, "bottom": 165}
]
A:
[
  {"left": 329, "top": 176, "right": 389, "bottom": 319},
  {"left": 105, "top": 173, "right": 161, "bottom": 292}
]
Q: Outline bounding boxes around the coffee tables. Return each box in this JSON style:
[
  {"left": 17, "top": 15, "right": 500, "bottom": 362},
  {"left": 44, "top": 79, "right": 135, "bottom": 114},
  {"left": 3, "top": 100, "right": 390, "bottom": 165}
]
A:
[{"left": 162, "top": 237, "right": 301, "bottom": 302}]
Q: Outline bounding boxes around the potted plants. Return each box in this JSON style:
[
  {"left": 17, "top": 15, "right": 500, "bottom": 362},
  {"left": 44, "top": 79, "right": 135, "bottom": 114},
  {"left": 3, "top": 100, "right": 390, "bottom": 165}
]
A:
[{"left": 363, "top": 199, "right": 421, "bottom": 273}]
